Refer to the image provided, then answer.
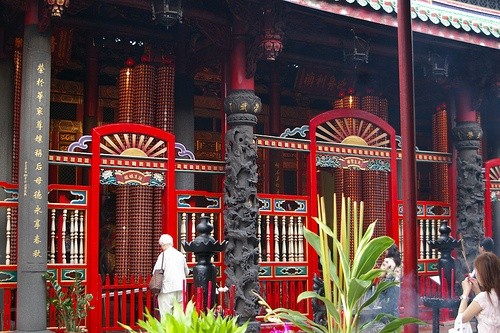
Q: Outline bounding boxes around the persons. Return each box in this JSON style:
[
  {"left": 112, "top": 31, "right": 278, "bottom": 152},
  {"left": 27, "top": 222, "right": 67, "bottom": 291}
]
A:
[
  {"left": 152, "top": 233, "right": 190, "bottom": 324},
  {"left": 468, "top": 238, "right": 495, "bottom": 278},
  {"left": 378, "top": 244, "right": 401, "bottom": 317},
  {"left": 455, "top": 252, "right": 500, "bottom": 333}
]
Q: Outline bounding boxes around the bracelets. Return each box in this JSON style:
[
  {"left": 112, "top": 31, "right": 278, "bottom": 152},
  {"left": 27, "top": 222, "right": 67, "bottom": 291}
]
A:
[{"left": 458, "top": 295, "right": 470, "bottom": 299}]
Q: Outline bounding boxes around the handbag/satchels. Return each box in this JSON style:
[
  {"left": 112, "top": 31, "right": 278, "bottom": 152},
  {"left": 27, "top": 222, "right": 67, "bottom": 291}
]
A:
[
  {"left": 149, "top": 251, "right": 165, "bottom": 291},
  {"left": 380, "top": 274, "right": 395, "bottom": 295}
]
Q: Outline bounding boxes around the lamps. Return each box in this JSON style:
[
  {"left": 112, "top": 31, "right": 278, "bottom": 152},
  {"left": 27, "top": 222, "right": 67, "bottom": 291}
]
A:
[
  {"left": 343, "top": 29, "right": 370, "bottom": 71},
  {"left": 151, "top": 0, "right": 183, "bottom": 30},
  {"left": 46, "top": 0, "right": 70, "bottom": 21},
  {"left": 431, "top": 55, "right": 449, "bottom": 86}
]
